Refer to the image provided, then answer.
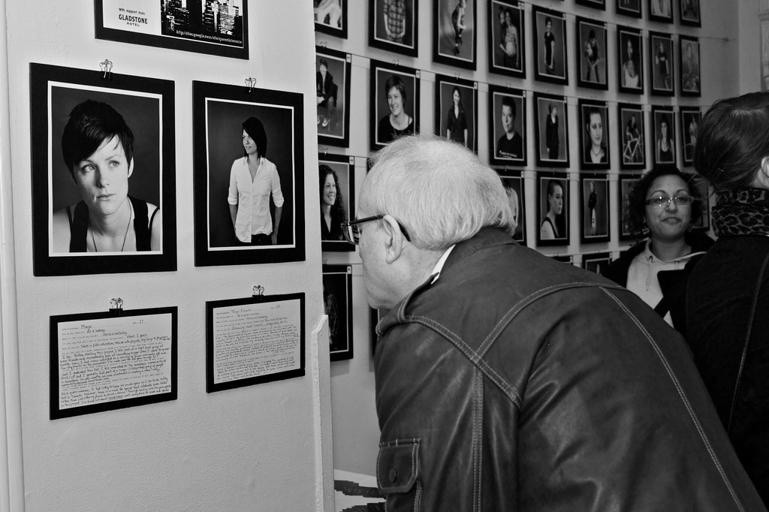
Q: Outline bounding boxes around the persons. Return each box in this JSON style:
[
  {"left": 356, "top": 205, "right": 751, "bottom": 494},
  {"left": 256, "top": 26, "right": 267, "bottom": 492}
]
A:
[
  {"left": 340, "top": 136, "right": 766, "bottom": 512},
  {"left": 53, "top": 98, "right": 160, "bottom": 253},
  {"left": 227, "top": 116, "right": 284, "bottom": 246},
  {"left": 314, "top": 1, "right": 702, "bottom": 353},
  {"left": 679, "top": 90, "right": 769, "bottom": 509}
]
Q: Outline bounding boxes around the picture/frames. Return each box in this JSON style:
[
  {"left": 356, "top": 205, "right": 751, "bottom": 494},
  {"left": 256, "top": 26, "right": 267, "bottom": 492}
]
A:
[
  {"left": 93, "top": 1, "right": 249, "bottom": 62},
  {"left": 30, "top": 62, "right": 177, "bottom": 276},
  {"left": 192, "top": 80, "right": 305, "bottom": 267},
  {"left": 205, "top": 292, "right": 306, "bottom": 395},
  {"left": 49, "top": 306, "right": 178, "bottom": 421}
]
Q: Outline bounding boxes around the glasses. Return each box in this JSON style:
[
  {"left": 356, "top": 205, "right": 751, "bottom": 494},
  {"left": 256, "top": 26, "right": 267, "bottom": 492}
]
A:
[
  {"left": 645, "top": 195, "right": 695, "bottom": 208},
  {"left": 340, "top": 213, "right": 413, "bottom": 245}
]
